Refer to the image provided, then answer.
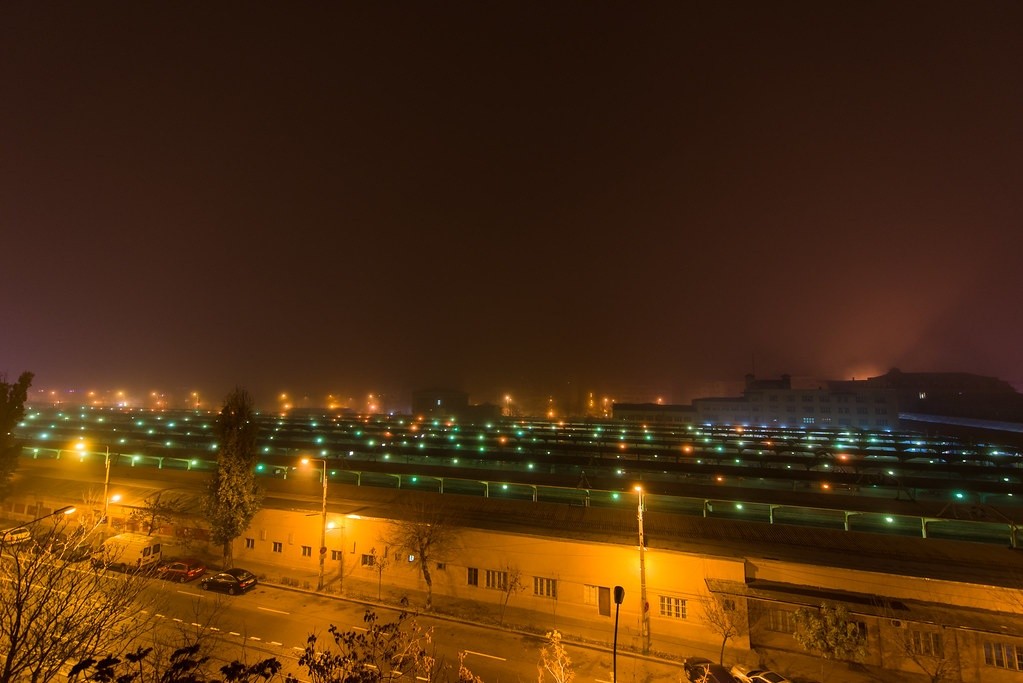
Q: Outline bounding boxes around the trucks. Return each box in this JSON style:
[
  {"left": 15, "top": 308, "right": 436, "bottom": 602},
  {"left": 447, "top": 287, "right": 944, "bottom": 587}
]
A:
[{"left": 89, "top": 533, "right": 161, "bottom": 574}]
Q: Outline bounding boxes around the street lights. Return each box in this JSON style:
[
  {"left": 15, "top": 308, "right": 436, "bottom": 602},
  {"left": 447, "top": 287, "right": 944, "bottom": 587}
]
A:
[
  {"left": 0, "top": 505, "right": 77, "bottom": 557},
  {"left": 634, "top": 485, "right": 652, "bottom": 655},
  {"left": 76, "top": 442, "right": 111, "bottom": 543},
  {"left": 300, "top": 458, "right": 328, "bottom": 589},
  {"left": 613, "top": 586, "right": 625, "bottom": 681}
]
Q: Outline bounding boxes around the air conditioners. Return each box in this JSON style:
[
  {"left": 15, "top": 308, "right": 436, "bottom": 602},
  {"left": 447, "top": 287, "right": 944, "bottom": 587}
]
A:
[{"left": 891, "top": 619, "right": 907, "bottom": 629}]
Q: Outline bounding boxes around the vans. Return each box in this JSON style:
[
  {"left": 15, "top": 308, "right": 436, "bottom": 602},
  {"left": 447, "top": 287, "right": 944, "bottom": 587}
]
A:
[
  {"left": 0, "top": 526, "right": 31, "bottom": 546},
  {"left": 26, "top": 532, "right": 67, "bottom": 553}
]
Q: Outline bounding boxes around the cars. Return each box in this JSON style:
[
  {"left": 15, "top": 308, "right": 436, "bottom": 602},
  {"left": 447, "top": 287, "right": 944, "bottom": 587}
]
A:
[
  {"left": 154, "top": 559, "right": 206, "bottom": 583},
  {"left": 55, "top": 541, "right": 93, "bottom": 563},
  {"left": 684, "top": 656, "right": 742, "bottom": 683},
  {"left": 201, "top": 568, "right": 257, "bottom": 594},
  {"left": 730, "top": 662, "right": 792, "bottom": 683}
]
